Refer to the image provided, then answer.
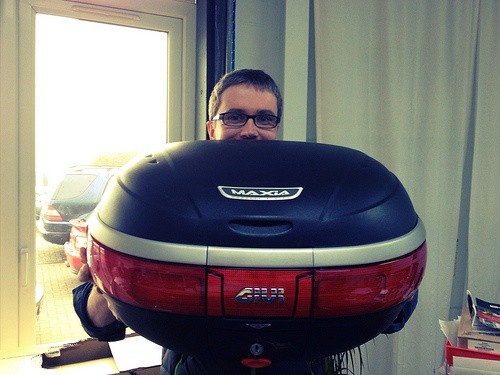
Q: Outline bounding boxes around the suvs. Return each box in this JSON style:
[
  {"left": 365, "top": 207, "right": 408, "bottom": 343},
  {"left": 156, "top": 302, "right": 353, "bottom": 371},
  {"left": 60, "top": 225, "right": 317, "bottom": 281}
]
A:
[{"left": 40, "top": 166, "right": 119, "bottom": 275}]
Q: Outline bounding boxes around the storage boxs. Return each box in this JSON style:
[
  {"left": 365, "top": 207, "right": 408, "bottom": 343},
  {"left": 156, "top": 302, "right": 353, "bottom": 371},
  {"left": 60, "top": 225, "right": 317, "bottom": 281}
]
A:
[{"left": 86, "top": 140, "right": 427, "bottom": 359}]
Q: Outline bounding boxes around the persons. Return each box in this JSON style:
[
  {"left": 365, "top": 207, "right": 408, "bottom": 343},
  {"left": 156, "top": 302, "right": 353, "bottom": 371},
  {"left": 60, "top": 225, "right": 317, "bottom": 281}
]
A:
[{"left": 72, "top": 69, "right": 418, "bottom": 375}]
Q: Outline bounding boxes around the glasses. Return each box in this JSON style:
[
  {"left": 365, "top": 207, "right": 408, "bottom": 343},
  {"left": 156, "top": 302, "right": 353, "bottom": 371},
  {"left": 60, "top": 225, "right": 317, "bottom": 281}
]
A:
[{"left": 212, "top": 112, "right": 281, "bottom": 129}]
